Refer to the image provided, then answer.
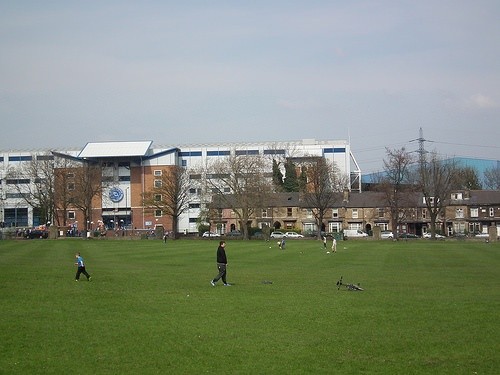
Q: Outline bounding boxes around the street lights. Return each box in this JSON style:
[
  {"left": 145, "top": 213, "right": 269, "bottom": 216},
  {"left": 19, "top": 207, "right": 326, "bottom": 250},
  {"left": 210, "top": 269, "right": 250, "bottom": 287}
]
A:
[{"left": 125, "top": 187, "right": 129, "bottom": 230}]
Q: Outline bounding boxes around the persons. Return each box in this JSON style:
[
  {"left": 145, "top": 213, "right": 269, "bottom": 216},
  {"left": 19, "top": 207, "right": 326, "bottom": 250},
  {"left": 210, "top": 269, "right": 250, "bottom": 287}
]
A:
[
  {"left": 183, "top": 230, "right": 187, "bottom": 236},
  {"left": 332, "top": 237, "right": 337, "bottom": 253},
  {"left": 322, "top": 235, "right": 327, "bottom": 249},
  {"left": 163, "top": 235, "right": 167, "bottom": 243},
  {"left": 277, "top": 238, "right": 285, "bottom": 249},
  {"left": 74, "top": 252, "right": 92, "bottom": 282},
  {"left": 145, "top": 228, "right": 155, "bottom": 239},
  {"left": 211, "top": 241, "right": 232, "bottom": 287},
  {"left": 95, "top": 226, "right": 138, "bottom": 236}
]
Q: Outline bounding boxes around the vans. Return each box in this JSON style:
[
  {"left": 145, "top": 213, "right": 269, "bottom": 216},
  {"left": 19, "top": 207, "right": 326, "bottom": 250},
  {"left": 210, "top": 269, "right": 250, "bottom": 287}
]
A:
[
  {"left": 381, "top": 231, "right": 394, "bottom": 239},
  {"left": 343, "top": 230, "right": 369, "bottom": 238}
]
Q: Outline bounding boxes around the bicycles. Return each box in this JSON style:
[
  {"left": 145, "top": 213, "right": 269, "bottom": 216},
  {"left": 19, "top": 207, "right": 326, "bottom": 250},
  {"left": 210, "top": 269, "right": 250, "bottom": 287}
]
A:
[{"left": 336, "top": 276, "right": 364, "bottom": 291}]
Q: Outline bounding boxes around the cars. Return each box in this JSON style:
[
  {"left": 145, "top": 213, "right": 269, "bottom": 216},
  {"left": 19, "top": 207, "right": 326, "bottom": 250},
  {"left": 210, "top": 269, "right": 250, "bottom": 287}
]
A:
[
  {"left": 475, "top": 232, "right": 490, "bottom": 237},
  {"left": 202, "top": 231, "right": 220, "bottom": 238},
  {"left": 286, "top": 232, "right": 304, "bottom": 239},
  {"left": 451, "top": 233, "right": 470, "bottom": 239},
  {"left": 398, "top": 232, "right": 420, "bottom": 238},
  {"left": 270, "top": 231, "right": 288, "bottom": 239},
  {"left": 229, "top": 230, "right": 241, "bottom": 237},
  {"left": 423, "top": 232, "right": 445, "bottom": 239},
  {"left": 24, "top": 230, "right": 49, "bottom": 239}
]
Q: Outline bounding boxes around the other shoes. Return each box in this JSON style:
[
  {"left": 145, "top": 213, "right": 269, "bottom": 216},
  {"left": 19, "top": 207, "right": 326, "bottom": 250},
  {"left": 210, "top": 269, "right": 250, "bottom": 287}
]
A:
[
  {"left": 210, "top": 280, "right": 215, "bottom": 287},
  {"left": 89, "top": 277, "right": 92, "bottom": 282},
  {"left": 224, "top": 283, "right": 231, "bottom": 286}
]
